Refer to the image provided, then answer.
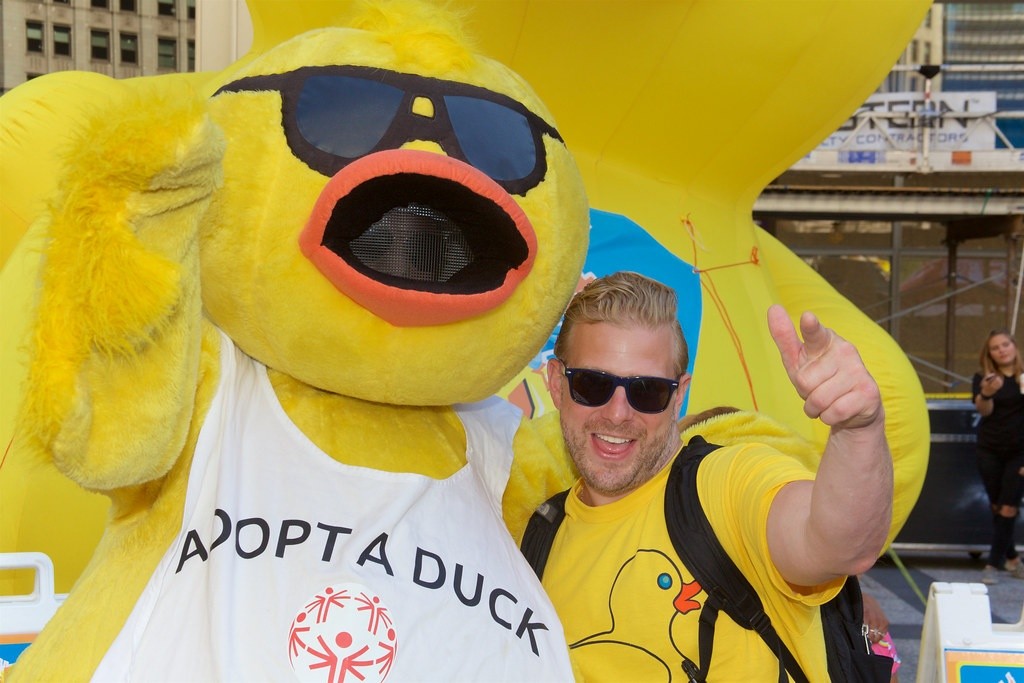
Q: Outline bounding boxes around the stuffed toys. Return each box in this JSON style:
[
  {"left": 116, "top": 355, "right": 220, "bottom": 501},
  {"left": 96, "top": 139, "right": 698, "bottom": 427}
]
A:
[{"left": 0, "top": 0, "right": 819, "bottom": 682}]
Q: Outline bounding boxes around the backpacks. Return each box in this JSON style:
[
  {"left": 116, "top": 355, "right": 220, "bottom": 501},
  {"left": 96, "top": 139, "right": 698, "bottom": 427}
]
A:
[{"left": 520, "top": 434, "right": 894, "bottom": 683}]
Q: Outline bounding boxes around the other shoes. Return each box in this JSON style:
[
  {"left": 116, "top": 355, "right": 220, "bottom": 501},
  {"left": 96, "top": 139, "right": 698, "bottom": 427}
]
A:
[
  {"left": 1005, "top": 560, "right": 1024, "bottom": 578},
  {"left": 982, "top": 566, "right": 997, "bottom": 584}
]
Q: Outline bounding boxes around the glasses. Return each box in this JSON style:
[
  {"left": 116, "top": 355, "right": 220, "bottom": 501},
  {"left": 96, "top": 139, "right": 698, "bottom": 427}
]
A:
[{"left": 564, "top": 368, "right": 679, "bottom": 412}]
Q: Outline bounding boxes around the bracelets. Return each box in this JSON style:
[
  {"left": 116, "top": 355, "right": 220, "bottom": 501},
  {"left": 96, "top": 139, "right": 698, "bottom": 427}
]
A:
[{"left": 980, "top": 392, "right": 993, "bottom": 400}]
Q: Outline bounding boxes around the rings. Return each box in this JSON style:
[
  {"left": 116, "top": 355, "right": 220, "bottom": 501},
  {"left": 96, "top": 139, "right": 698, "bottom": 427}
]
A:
[{"left": 870, "top": 629, "right": 884, "bottom": 636}]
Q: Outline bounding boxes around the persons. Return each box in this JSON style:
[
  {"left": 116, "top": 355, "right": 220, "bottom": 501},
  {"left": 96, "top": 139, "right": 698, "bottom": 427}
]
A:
[
  {"left": 520, "top": 270, "right": 900, "bottom": 683},
  {"left": 972, "top": 330, "right": 1024, "bottom": 571}
]
